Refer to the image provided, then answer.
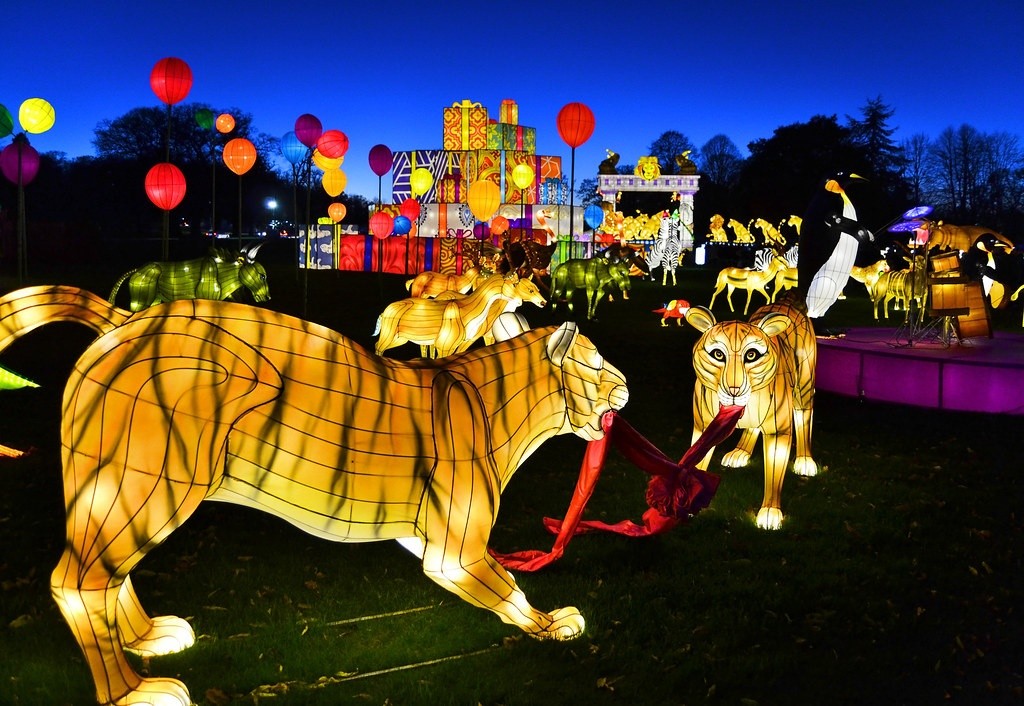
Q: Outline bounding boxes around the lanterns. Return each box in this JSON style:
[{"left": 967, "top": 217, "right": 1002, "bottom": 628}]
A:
[
  {"left": 328, "top": 203, "right": 346, "bottom": 223},
  {"left": 511, "top": 164, "right": 535, "bottom": 188},
  {"left": 195, "top": 109, "right": 214, "bottom": 129},
  {"left": 401, "top": 198, "right": 420, "bottom": 220},
  {"left": 0, "top": 103, "right": 14, "bottom": 138},
  {"left": 371, "top": 212, "right": 394, "bottom": 239},
  {"left": 295, "top": 114, "right": 322, "bottom": 147},
  {"left": 215, "top": 114, "right": 236, "bottom": 134},
  {"left": 468, "top": 180, "right": 500, "bottom": 222},
  {"left": 393, "top": 216, "right": 412, "bottom": 234},
  {"left": 557, "top": 102, "right": 595, "bottom": 148},
  {"left": 316, "top": 129, "right": 349, "bottom": 159},
  {"left": 145, "top": 162, "right": 186, "bottom": 210},
  {"left": 0, "top": 141, "right": 40, "bottom": 187},
  {"left": 150, "top": 56, "right": 194, "bottom": 106},
  {"left": 282, "top": 130, "right": 308, "bottom": 165},
  {"left": 584, "top": 205, "right": 603, "bottom": 229},
  {"left": 322, "top": 168, "right": 347, "bottom": 197},
  {"left": 19, "top": 98, "right": 55, "bottom": 135},
  {"left": 491, "top": 217, "right": 509, "bottom": 235},
  {"left": 222, "top": 138, "right": 257, "bottom": 175},
  {"left": 370, "top": 144, "right": 393, "bottom": 176},
  {"left": 410, "top": 168, "right": 434, "bottom": 196},
  {"left": 473, "top": 224, "right": 490, "bottom": 240},
  {"left": 401, "top": 221, "right": 417, "bottom": 239},
  {"left": 313, "top": 147, "right": 344, "bottom": 170}
]
